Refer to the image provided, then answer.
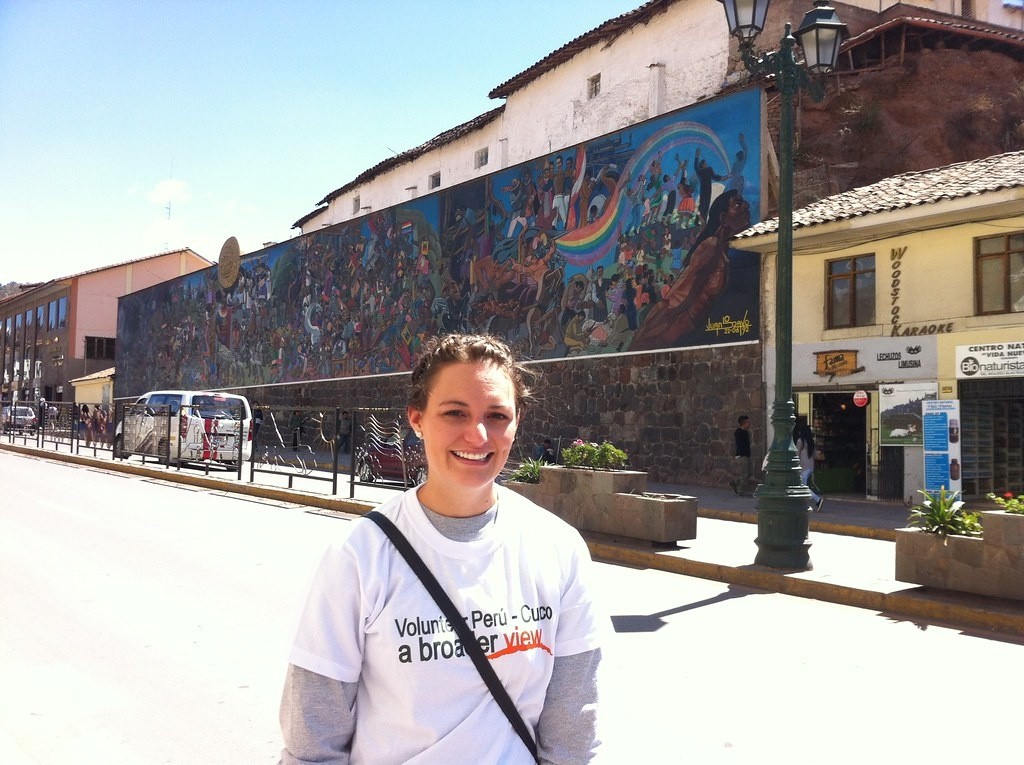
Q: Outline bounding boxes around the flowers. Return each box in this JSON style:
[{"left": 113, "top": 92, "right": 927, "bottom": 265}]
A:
[
  {"left": 985, "top": 492, "right": 1024, "bottom": 514},
  {"left": 559, "top": 439, "right": 628, "bottom": 471}
]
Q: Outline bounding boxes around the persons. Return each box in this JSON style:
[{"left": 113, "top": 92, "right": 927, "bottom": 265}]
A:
[
  {"left": 287, "top": 410, "right": 305, "bottom": 452},
  {"left": 537, "top": 439, "right": 552, "bottom": 463},
  {"left": 337, "top": 411, "right": 352, "bottom": 455},
  {"left": 276, "top": 332, "right": 621, "bottom": 764},
  {"left": 796, "top": 424, "right": 824, "bottom": 512},
  {"left": 40, "top": 398, "right": 117, "bottom": 445},
  {"left": 729, "top": 415, "right": 752, "bottom": 497},
  {"left": 888, "top": 423, "right": 916, "bottom": 439},
  {"left": 250, "top": 401, "right": 264, "bottom": 450}
]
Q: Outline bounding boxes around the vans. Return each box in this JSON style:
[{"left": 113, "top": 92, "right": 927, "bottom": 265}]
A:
[{"left": 115, "top": 389, "right": 253, "bottom": 471}]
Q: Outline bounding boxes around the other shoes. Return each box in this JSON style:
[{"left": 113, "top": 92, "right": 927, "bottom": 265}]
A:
[
  {"left": 817, "top": 499, "right": 824, "bottom": 512},
  {"left": 729, "top": 482, "right": 737, "bottom": 494}
]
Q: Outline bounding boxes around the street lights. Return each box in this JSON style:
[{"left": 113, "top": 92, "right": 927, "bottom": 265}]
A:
[{"left": 724, "top": 1, "right": 845, "bottom": 569}]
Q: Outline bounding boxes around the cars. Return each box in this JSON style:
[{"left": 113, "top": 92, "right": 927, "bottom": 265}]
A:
[
  {"left": 355, "top": 424, "right": 428, "bottom": 486},
  {"left": 883, "top": 412, "right": 922, "bottom": 428}
]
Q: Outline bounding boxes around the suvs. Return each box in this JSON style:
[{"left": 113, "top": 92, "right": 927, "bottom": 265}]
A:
[{"left": 1, "top": 406, "right": 38, "bottom": 436}]
